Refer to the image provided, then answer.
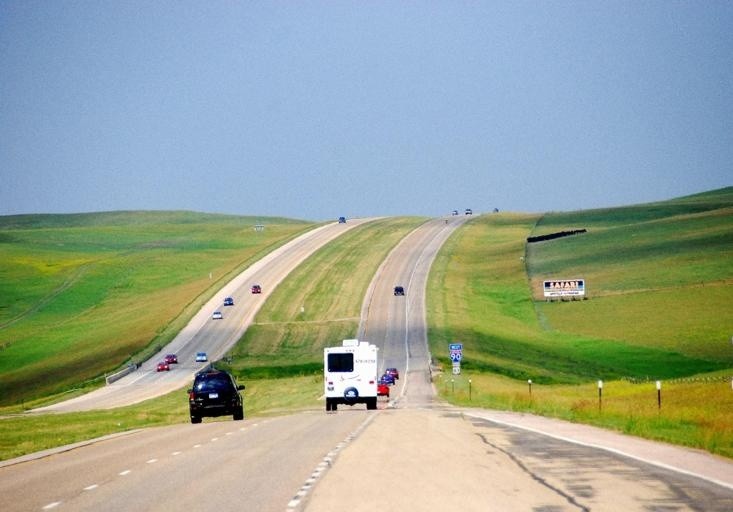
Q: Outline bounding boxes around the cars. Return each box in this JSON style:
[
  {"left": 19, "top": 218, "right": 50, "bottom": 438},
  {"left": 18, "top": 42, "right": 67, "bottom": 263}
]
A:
[
  {"left": 224, "top": 297, "right": 233, "bottom": 306},
  {"left": 213, "top": 311, "right": 222, "bottom": 319},
  {"left": 377, "top": 366, "right": 398, "bottom": 397},
  {"left": 157, "top": 361, "right": 170, "bottom": 372},
  {"left": 394, "top": 286, "right": 404, "bottom": 296},
  {"left": 196, "top": 352, "right": 207, "bottom": 362},
  {"left": 466, "top": 209, "right": 472, "bottom": 215},
  {"left": 252, "top": 285, "right": 261, "bottom": 293},
  {"left": 452, "top": 211, "right": 458, "bottom": 216},
  {"left": 164, "top": 353, "right": 177, "bottom": 364}
]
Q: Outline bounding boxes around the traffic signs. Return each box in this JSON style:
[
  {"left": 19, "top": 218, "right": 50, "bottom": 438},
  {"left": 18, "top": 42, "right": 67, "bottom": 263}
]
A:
[{"left": 450, "top": 352, "right": 462, "bottom": 362}]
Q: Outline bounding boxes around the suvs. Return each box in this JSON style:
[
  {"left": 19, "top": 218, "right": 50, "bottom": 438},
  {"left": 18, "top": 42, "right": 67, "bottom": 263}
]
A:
[
  {"left": 339, "top": 217, "right": 346, "bottom": 223},
  {"left": 187, "top": 365, "right": 246, "bottom": 424}
]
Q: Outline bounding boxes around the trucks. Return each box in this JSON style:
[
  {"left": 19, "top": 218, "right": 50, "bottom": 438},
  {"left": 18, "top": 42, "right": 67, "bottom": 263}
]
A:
[{"left": 323, "top": 338, "right": 379, "bottom": 411}]
